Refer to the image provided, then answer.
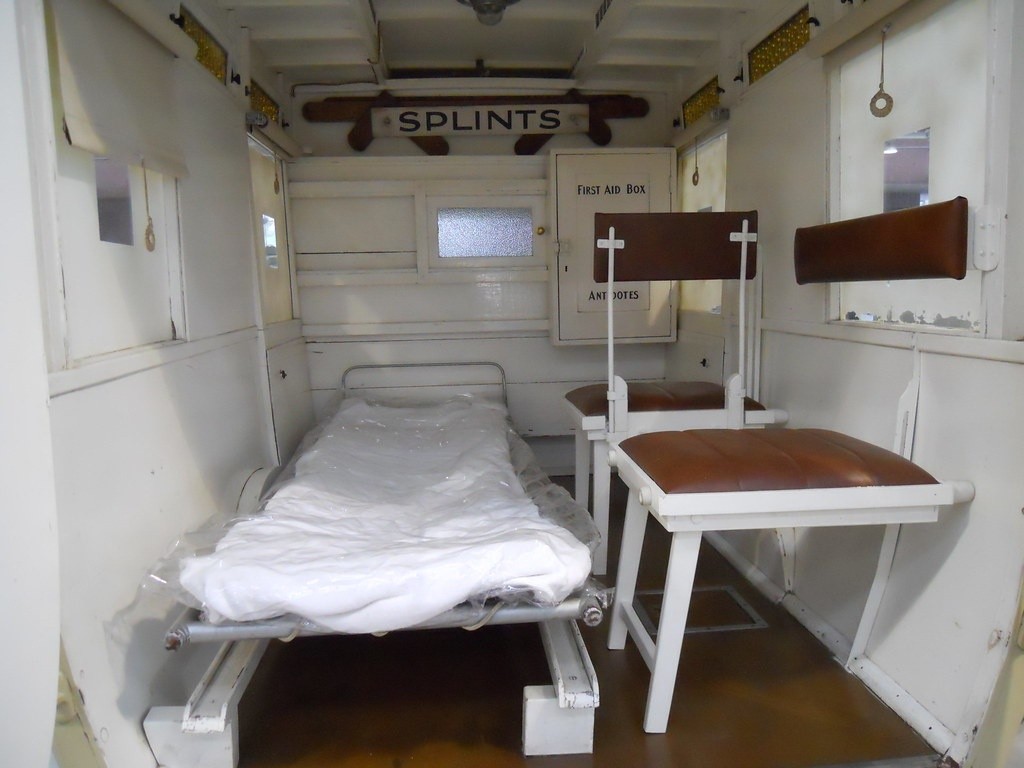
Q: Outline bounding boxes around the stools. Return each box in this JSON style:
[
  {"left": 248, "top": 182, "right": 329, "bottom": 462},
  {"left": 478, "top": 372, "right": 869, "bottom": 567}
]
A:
[
  {"left": 608, "top": 430, "right": 976, "bottom": 734},
  {"left": 564, "top": 382, "right": 789, "bottom": 576}
]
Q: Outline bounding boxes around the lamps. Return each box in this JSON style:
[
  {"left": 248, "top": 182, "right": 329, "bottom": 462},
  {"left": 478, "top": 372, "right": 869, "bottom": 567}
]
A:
[{"left": 457, "top": 0, "right": 522, "bottom": 25}]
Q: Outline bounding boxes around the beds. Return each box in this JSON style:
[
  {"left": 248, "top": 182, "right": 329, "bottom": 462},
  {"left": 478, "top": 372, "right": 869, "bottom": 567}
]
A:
[{"left": 164, "top": 360, "right": 603, "bottom": 735}]
